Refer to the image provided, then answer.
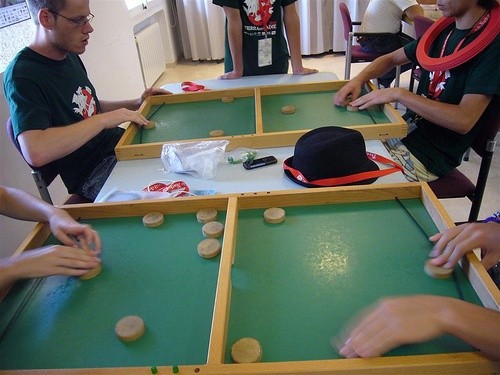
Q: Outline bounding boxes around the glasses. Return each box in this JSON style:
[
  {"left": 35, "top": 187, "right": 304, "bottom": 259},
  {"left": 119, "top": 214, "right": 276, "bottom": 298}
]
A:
[{"left": 46, "top": 9, "right": 95, "bottom": 28}]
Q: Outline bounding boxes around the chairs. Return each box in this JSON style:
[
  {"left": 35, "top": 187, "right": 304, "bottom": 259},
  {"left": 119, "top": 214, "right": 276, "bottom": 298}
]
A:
[
  {"left": 406, "top": 16, "right": 437, "bottom": 111},
  {"left": 338, "top": 1, "right": 403, "bottom": 111},
  {"left": 7, "top": 115, "right": 96, "bottom": 205},
  {"left": 425, "top": 106, "right": 500, "bottom": 228}
]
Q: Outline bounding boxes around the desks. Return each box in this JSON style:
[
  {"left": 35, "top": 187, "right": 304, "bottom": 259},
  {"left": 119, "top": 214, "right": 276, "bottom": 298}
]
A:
[
  {"left": 400, "top": 3, "right": 447, "bottom": 40},
  {"left": 89, "top": 72, "right": 414, "bottom": 203}
]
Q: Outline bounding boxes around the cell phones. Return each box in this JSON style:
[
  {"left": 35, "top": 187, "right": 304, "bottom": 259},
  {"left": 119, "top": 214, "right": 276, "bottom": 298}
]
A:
[{"left": 243, "top": 156, "right": 277, "bottom": 170}]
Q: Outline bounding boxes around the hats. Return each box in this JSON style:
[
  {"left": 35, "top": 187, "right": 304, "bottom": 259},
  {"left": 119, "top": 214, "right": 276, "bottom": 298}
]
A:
[{"left": 282, "top": 126, "right": 380, "bottom": 189}]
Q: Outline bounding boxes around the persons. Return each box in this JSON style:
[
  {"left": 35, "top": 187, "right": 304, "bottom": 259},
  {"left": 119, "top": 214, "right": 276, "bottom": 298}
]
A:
[
  {"left": 1, "top": 186, "right": 102, "bottom": 298},
  {"left": 329, "top": 0, "right": 500, "bottom": 179},
  {"left": 336, "top": 211, "right": 500, "bottom": 359},
  {"left": 357, "top": 0, "right": 426, "bottom": 92},
  {"left": 3, "top": 0, "right": 175, "bottom": 198},
  {"left": 212, "top": 0, "right": 320, "bottom": 80}
]
